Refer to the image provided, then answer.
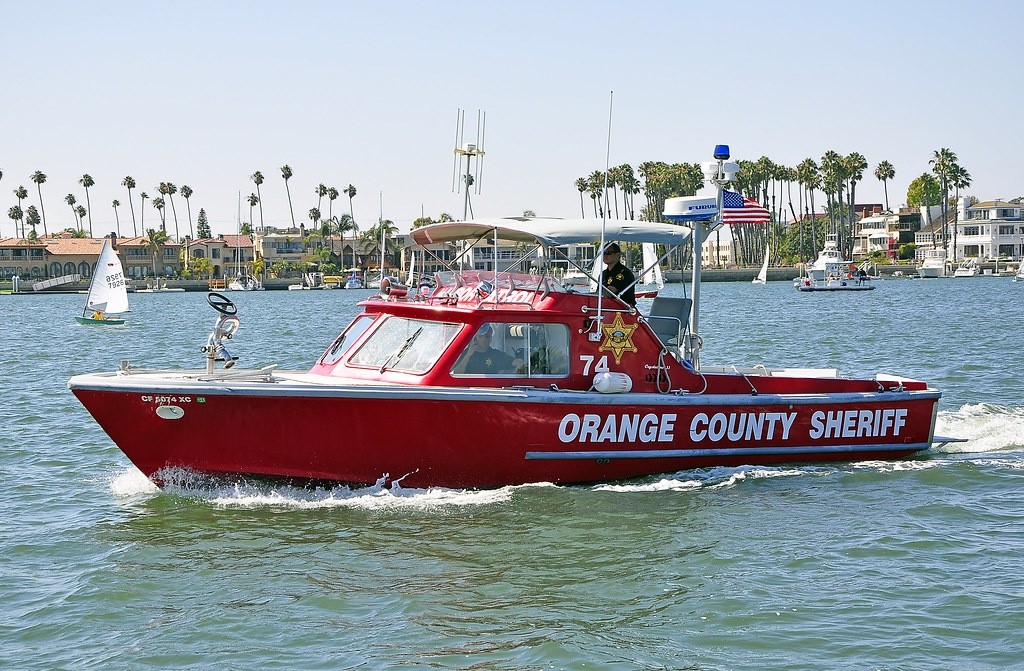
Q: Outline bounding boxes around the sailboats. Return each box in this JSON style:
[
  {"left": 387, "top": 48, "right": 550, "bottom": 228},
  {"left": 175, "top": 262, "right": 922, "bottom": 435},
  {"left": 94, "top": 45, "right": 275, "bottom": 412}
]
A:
[
  {"left": 228, "top": 191, "right": 256, "bottom": 291},
  {"left": 633, "top": 241, "right": 666, "bottom": 298},
  {"left": 341, "top": 227, "right": 364, "bottom": 290},
  {"left": 416, "top": 204, "right": 434, "bottom": 288},
  {"left": 74, "top": 238, "right": 132, "bottom": 326},
  {"left": 581, "top": 240, "right": 609, "bottom": 294},
  {"left": 751, "top": 241, "right": 770, "bottom": 285},
  {"left": 369, "top": 228, "right": 389, "bottom": 290}
]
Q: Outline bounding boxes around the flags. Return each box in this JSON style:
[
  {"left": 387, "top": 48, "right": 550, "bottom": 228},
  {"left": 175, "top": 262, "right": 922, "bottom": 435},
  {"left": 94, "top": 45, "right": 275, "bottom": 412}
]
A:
[{"left": 722, "top": 188, "right": 771, "bottom": 224}]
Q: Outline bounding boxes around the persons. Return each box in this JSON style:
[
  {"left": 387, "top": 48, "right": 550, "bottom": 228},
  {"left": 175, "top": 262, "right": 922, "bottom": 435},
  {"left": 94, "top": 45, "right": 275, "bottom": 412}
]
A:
[
  {"left": 888, "top": 236, "right": 902, "bottom": 265},
  {"left": 453, "top": 324, "right": 525, "bottom": 374},
  {"left": 597, "top": 243, "right": 637, "bottom": 307},
  {"left": 91, "top": 310, "right": 103, "bottom": 320},
  {"left": 859, "top": 268, "right": 867, "bottom": 286}
]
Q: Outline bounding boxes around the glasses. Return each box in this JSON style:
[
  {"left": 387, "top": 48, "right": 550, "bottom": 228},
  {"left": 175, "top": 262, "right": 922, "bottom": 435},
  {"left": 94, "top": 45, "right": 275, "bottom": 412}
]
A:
[
  {"left": 604, "top": 251, "right": 618, "bottom": 255},
  {"left": 477, "top": 333, "right": 491, "bottom": 338}
]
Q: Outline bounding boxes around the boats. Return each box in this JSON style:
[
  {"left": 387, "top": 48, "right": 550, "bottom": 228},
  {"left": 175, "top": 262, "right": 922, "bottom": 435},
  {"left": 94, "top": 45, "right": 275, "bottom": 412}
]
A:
[
  {"left": 1013, "top": 253, "right": 1024, "bottom": 282},
  {"left": 915, "top": 232, "right": 952, "bottom": 279},
  {"left": 953, "top": 257, "right": 982, "bottom": 277},
  {"left": 561, "top": 267, "right": 590, "bottom": 285},
  {"left": 793, "top": 260, "right": 877, "bottom": 292},
  {"left": 66, "top": 90, "right": 973, "bottom": 494},
  {"left": 806, "top": 220, "right": 845, "bottom": 281}
]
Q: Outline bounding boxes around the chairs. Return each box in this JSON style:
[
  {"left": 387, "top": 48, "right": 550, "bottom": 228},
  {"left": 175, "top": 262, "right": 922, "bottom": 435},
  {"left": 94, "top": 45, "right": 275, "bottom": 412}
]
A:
[{"left": 648, "top": 297, "right": 693, "bottom": 351}]
